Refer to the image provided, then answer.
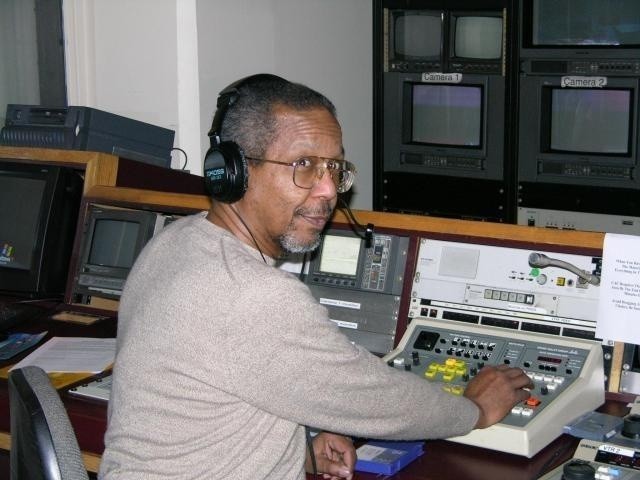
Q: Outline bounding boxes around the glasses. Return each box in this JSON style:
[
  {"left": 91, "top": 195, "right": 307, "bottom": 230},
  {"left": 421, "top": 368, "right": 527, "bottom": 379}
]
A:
[{"left": 244, "top": 152, "right": 359, "bottom": 196}]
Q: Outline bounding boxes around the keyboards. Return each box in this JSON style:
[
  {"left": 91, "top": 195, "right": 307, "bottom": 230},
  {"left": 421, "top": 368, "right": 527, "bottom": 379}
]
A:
[{"left": 0, "top": 302, "right": 39, "bottom": 327}]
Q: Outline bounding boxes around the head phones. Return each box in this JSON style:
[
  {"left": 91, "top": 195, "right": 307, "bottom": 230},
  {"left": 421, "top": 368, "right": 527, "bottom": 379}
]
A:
[{"left": 203, "top": 73, "right": 353, "bottom": 209}]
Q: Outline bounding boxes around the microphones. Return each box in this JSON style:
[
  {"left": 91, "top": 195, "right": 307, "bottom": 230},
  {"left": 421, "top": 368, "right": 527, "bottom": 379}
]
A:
[
  {"left": 527, "top": 253, "right": 601, "bottom": 286},
  {"left": 339, "top": 198, "right": 375, "bottom": 248}
]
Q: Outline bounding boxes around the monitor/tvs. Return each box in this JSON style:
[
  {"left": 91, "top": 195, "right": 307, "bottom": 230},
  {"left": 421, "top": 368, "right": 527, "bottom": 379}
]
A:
[
  {"left": 0, "top": 162, "right": 85, "bottom": 300},
  {"left": 387, "top": 7, "right": 448, "bottom": 73},
  {"left": 446, "top": 10, "right": 506, "bottom": 75},
  {"left": 72, "top": 203, "right": 157, "bottom": 301},
  {"left": 516, "top": 73, "right": 640, "bottom": 211},
  {"left": 518, "top": 0, "right": 640, "bottom": 77},
  {"left": 383, "top": 71, "right": 506, "bottom": 182}
]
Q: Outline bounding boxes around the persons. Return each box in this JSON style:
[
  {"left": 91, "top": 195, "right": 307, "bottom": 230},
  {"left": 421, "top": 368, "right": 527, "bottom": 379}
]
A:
[{"left": 97, "top": 73, "right": 536, "bottom": 480}]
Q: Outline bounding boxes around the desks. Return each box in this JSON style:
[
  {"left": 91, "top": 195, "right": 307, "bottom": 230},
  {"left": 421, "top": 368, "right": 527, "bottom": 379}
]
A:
[{"left": 0, "top": 360, "right": 640, "bottom": 480}]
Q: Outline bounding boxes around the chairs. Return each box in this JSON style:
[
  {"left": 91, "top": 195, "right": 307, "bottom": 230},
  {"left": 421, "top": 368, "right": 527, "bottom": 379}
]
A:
[{"left": 6, "top": 364, "right": 91, "bottom": 480}]
